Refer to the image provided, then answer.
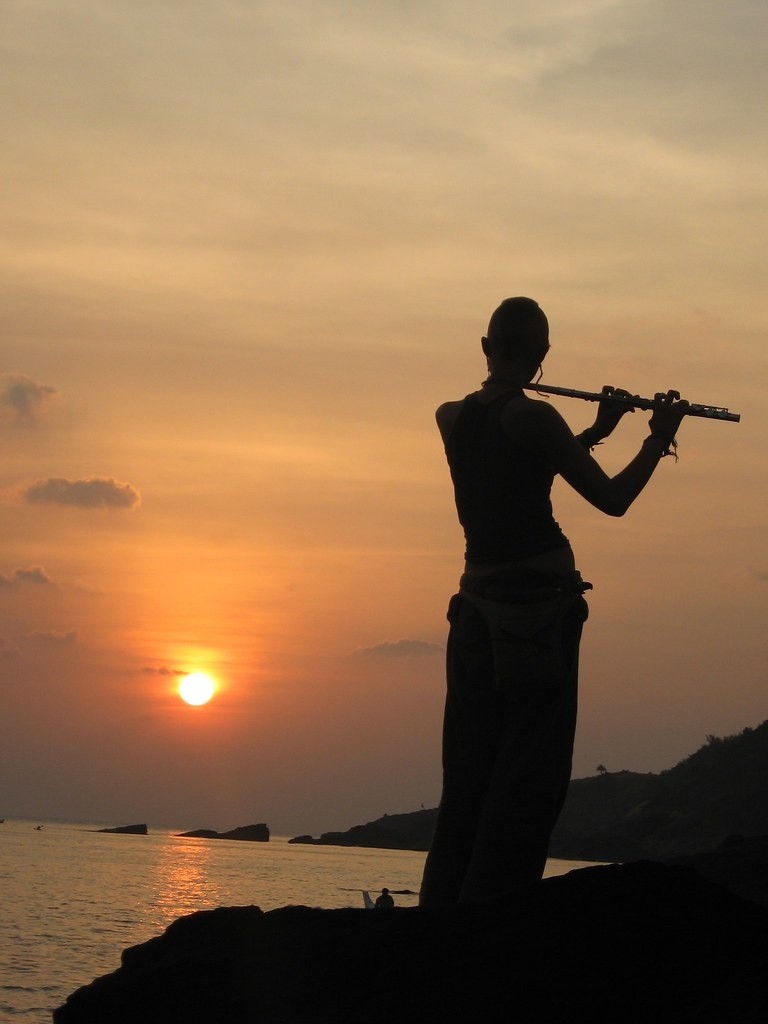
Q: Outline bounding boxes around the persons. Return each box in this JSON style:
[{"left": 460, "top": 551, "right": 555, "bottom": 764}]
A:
[
  {"left": 374, "top": 888, "right": 394, "bottom": 909},
  {"left": 418, "top": 295, "right": 689, "bottom": 906}
]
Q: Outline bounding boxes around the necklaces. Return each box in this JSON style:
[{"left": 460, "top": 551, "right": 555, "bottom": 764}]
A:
[{"left": 482, "top": 375, "right": 525, "bottom": 390}]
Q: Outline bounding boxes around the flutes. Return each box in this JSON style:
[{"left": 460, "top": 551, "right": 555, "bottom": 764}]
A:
[{"left": 517, "top": 379, "right": 742, "bottom": 423}]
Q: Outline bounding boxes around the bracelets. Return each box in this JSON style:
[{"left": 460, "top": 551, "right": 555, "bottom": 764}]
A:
[
  {"left": 574, "top": 427, "right": 603, "bottom": 452},
  {"left": 642, "top": 430, "right": 678, "bottom": 462}
]
